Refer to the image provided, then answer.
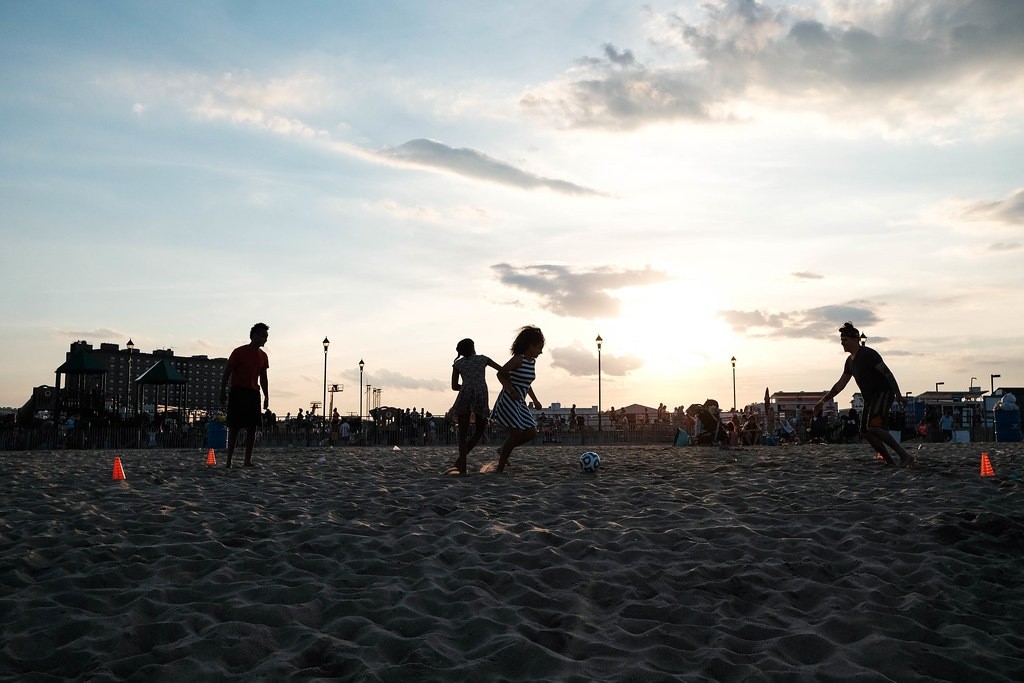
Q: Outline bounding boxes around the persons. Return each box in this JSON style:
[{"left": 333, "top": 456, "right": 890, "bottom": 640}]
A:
[
  {"left": 814, "top": 324, "right": 914, "bottom": 467},
  {"left": 939, "top": 410, "right": 953, "bottom": 441},
  {"left": 538, "top": 400, "right": 684, "bottom": 443},
  {"left": 491, "top": 327, "right": 544, "bottom": 475},
  {"left": 725, "top": 402, "right": 863, "bottom": 446},
  {"left": 66, "top": 419, "right": 74, "bottom": 434},
  {"left": 741, "top": 416, "right": 762, "bottom": 445},
  {"left": 181, "top": 405, "right": 495, "bottom": 446},
  {"left": 447, "top": 339, "right": 502, "bottom": 477},
  {"left": 219, "top": 323, "right": 269, "bottom": 469}
]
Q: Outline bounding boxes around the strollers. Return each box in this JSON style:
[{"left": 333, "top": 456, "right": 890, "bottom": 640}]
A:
[
  {"left": 778, "top": 419, "right": 799, "bottom": 446},
  {"left": 693, "top": 406, "right": 728, "bottom": 446}
]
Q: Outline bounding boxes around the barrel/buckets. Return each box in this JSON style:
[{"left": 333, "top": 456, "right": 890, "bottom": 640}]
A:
[
  {"left": 206, "top": 422, "right": 227, "bottom": 449},
  {"left": 994, "top": 409, "right": 1021, "bottom": 442}
]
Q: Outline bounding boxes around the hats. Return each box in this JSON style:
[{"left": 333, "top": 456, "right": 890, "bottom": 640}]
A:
[{"left": 572, "top": 404, "right": 576, "bottom": 406}]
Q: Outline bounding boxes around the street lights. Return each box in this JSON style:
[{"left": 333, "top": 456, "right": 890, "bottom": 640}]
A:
[
  {"left": 906, "top": 391, "right": 912, "bottom": 397},
  {"left": 969, "top": 376, "right": 979, "bottom": 388},
  {"left": 322, "top": 334, "right": 330, "bottom": 444},
  {"left": 935, "top": 381, "right": 946, "bottom": 391},
  {"left": 990, "top": 373, "right": 1001, "bottom": 396},
  {"left": 591, "top": 332, "right": 607, "bottom": 430},
  {"left": 729, "top": 355, "right": 738, "bottom": 412},
  {"left": 126, "top": 337, "right": 134, "bottom": 422},
  {"left": 357, "top": 359, "right": 365, "bottom": 419}
]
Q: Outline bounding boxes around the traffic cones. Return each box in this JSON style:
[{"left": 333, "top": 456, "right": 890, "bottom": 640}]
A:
[
  {"left": 112, "top": 456, "right": 127, "bottom": 480},
  {"left": 980, "top": 453, "right": 995, "bottom": 478},
  {"left": 206, "top": 448, "right": 218, "bottom": 465}
]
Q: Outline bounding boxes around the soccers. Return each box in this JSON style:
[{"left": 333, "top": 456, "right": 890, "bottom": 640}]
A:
[{"left": 579, "top": 450, "right": 602, "bottom": 473}]
